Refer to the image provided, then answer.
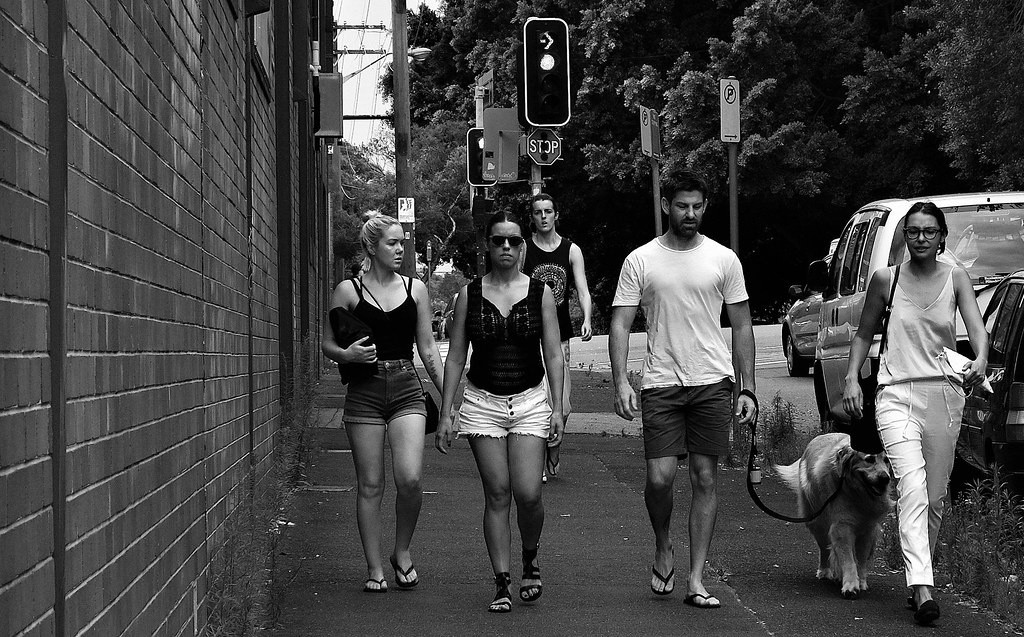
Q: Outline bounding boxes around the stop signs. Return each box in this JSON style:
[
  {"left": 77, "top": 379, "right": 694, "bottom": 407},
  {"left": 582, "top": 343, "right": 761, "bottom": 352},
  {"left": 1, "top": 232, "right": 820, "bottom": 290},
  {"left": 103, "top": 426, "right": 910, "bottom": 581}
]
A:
[{"left": 527, "top": 128, "right": 562, "bottom": 165}]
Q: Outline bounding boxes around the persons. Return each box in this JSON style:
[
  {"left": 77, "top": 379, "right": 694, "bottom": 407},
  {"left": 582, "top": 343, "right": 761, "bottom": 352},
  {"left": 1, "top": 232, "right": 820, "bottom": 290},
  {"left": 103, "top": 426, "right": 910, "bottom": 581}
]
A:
[
  {"left": 322, "top": 215, "right": 456, "bottom": 592},
  {"left": 435, "top": 214, "right": 565, "bottom": 612},
  {"left": 517, "top": 195, "right": 591, "bottom": 482},
  {"left": 608, "top": 181, "right": 757, "bottom": 607},
  {"left": 843, "top": 203, "right": 988, "bottom": 623}
]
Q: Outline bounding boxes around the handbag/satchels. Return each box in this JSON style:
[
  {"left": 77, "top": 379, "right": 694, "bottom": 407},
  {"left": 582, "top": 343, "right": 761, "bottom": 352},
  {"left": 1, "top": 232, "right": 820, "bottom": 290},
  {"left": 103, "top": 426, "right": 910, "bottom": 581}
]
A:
[
  {"left": 850, "top": 374, "right": 883, "bottom": 452},
  {"left": 938, "top": 346, "right": 994, "bottom": 398},
  {"left": 423, "top": 392, "right": 439, "bottom": 435}
]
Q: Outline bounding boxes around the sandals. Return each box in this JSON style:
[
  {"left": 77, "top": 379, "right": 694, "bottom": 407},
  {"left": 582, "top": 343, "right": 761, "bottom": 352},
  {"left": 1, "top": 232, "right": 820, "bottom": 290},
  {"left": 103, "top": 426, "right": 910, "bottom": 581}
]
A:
[
  {"left": 519, "top": 544, "right": 543, "bottom": 601},
  {"left": 487, "top": 572, "right": 512, "bottom": 613}
]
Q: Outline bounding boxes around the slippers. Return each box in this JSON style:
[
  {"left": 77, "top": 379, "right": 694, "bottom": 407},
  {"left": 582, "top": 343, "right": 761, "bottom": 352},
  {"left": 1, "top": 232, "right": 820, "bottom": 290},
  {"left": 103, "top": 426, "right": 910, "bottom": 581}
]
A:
[
  {"left": 684, "top": 592, "right": 721, "bottom": 608},
  {"left": 651, "top": 548, "right": 675, "bottom": 595},
  {"left": 389, "top": 555, "right": 419, "bottom": 587},
  {"left": 363, "top": 578, "right": 388, "bottom": 592},
  {"left": 547, "top": 446, "right": 559, "bottom": 475}
]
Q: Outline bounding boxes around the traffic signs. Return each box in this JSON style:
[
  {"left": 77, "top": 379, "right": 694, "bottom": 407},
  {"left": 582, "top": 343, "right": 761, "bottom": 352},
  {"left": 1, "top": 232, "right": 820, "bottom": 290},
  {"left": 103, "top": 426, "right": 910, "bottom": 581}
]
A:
[
  {"left": 720, "top": 80, "right": 741, "bottom": 142},
  {"left": 639, "top": 105, "right": 663, "bottom": 161}
]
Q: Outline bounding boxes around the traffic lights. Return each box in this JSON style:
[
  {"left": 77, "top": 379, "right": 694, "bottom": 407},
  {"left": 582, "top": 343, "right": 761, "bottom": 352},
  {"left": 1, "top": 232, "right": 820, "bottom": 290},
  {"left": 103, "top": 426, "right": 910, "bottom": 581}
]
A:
[
  {"left": 467, "top": 128, "right": 497, "bottom": 187},
  {"left": 524, "top": 17, "right": 572, "bottom": 128}
]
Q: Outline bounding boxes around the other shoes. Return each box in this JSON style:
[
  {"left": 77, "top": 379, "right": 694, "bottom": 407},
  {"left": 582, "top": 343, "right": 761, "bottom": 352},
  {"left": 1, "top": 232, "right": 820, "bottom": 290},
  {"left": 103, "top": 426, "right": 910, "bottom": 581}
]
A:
[{"left": 913, "top": 592, "right": 939, "bottom": 621}]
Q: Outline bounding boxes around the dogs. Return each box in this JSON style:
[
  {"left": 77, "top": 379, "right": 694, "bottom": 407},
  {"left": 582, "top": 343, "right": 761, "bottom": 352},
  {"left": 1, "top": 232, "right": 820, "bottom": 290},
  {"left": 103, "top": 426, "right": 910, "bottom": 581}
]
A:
[{"left": 772, "top": 433, "right": 898, "bottom": 599}]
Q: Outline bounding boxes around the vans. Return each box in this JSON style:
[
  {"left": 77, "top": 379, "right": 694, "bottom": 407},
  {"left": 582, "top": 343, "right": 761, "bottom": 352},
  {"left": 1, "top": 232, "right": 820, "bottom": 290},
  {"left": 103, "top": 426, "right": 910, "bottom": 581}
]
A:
[
  {"left": 807, "top": 191, "right": 1024, "bottom": 434},
  {"left": 438, "top": 293, "right": 458, "bottom": 340}
]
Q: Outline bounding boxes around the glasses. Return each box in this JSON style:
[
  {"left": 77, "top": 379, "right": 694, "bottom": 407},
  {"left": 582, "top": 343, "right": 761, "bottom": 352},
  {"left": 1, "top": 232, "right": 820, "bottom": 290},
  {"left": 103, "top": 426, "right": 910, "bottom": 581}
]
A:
[
  {"left": 488, "top": 235, "right": 523, "bottom": 246},
  {"left": 903, "top": 227, "right": 942, "bottom": 240}
]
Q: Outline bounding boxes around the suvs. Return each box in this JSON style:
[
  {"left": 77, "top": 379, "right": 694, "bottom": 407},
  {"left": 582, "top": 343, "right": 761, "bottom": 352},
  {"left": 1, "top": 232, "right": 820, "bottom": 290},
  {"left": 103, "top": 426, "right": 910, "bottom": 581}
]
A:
[
  {"left": 782, "top": 253, "right": 834, "bottom": 377},
  {"left": 955, "top": 270, "right": 1024, "bottom": 490}
]
